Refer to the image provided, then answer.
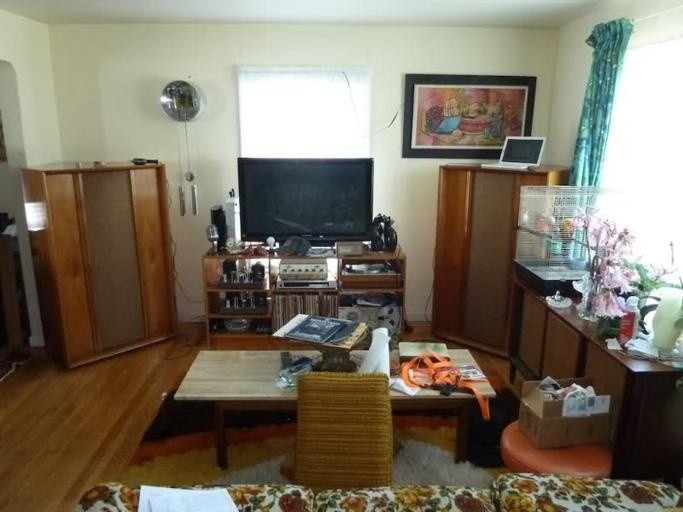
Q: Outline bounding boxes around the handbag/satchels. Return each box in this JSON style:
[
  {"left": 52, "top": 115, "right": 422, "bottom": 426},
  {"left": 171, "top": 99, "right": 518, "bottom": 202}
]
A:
[{"left": 466, "top": 387, "right": 518, "bottom": 468}]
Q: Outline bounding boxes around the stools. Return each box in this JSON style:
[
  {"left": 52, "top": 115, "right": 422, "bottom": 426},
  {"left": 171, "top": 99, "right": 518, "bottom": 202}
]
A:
[{"left": 499, "top": 419, "right": 612, "bottom": 478}]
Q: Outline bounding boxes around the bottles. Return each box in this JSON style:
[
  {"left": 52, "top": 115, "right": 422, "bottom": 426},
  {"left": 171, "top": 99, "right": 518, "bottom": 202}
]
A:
[{"left": 618, "top": 296, "right": 641, "bottom": 346}]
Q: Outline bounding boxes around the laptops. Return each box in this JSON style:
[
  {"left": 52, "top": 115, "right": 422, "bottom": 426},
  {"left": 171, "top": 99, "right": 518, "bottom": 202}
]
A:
[{"left": 480, "top": 136, "right": 548, "bottom": 170}]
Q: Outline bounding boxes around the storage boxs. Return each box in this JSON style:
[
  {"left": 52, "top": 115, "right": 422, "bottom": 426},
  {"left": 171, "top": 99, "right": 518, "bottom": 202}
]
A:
[{"left": 519, "top": 377, "right": 618, "bottom": 450}]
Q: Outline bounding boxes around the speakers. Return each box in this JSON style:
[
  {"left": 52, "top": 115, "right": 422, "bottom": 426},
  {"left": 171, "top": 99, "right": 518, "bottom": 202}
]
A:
[{"left": 211, "top": 205, "right": 227, "bottom": 251}]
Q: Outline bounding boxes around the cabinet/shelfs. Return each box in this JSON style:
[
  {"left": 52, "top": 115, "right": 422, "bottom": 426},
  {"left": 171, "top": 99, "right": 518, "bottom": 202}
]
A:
[
  {"left": 515, "top": 290, "right": 683, "bottom": 480},
  {"left": 23, "top": 167, "right": 182, "bottom": 369},
  {"left": 202, "top": 259, "right": 406, "bottom": 349},
  {"left": 433, "top": 170, "right": 569, "bottom": 358}
]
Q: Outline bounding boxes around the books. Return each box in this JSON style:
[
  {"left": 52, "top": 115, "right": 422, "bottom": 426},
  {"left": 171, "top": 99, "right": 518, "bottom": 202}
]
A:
[{"left": 398, "top": 341, "right": 450, "bottom": 361}]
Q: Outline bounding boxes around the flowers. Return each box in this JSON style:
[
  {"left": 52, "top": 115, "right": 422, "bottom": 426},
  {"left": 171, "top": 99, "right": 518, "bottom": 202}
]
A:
[{"left": 571, "top": 206, "right": 667, "bottom": 320}]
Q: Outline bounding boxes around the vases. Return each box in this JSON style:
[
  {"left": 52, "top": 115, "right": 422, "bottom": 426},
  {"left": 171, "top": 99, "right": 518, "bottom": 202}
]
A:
[{"left": 572, "top": 274, "right": 603, "bottom": 322}]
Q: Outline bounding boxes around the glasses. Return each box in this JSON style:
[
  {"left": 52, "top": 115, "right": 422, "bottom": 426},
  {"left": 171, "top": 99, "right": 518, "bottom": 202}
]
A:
[{"left": 276, "top": 236, "right": 310, "bottom": 255}]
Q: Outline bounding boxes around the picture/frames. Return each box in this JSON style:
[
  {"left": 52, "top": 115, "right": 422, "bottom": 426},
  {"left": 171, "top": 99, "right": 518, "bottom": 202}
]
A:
[{"left": 402, "top": 73, "right": 537, "bottom": 159}]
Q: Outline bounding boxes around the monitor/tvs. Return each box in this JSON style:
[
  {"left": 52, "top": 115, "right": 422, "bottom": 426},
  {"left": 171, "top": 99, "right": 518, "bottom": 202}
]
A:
[{"left": 237, "top": 157, "right": 374, "bottom": 249}]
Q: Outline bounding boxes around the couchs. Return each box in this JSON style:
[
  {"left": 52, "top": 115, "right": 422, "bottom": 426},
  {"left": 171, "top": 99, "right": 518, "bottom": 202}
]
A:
[{"left": 78, "top": 473, "right": 681, "bottom": 511}]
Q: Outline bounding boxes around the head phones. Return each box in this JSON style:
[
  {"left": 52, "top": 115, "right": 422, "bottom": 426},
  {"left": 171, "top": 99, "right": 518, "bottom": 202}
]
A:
[{"left": 369, "top": 216, "right": 397, "bottom": 252}]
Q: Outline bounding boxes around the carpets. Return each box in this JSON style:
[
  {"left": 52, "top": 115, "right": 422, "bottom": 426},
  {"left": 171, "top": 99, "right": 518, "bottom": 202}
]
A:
[{"left": 122, "top": 387, "right": 520, "bottom": 483}]
